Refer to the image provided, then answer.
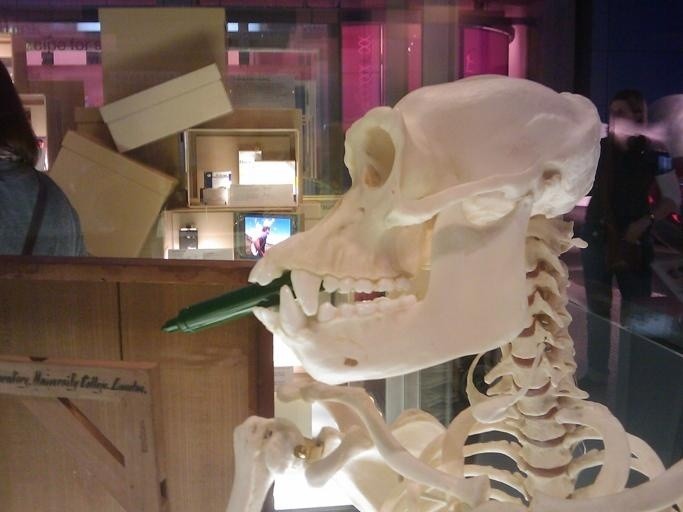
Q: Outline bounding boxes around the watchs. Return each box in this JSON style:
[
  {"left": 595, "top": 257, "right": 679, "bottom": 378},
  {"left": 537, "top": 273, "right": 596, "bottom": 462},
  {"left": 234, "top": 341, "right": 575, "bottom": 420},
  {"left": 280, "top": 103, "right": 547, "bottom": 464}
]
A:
[{"left": 646, "top": 211, "right": 656, "bottom": 224}]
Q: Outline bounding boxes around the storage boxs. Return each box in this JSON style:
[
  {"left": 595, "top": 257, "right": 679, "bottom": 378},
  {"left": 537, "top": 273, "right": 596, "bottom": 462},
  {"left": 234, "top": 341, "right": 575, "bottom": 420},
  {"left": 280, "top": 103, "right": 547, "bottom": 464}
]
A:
[{"left": 43, "top": 59, "right": 305, "bottom": 270}]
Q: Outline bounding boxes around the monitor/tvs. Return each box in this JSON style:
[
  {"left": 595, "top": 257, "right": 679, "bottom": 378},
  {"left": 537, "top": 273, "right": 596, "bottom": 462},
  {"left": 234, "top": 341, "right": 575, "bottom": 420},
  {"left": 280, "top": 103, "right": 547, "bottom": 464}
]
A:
[{"left": 234, "top": 211, "right": 305, "bottom": 262}]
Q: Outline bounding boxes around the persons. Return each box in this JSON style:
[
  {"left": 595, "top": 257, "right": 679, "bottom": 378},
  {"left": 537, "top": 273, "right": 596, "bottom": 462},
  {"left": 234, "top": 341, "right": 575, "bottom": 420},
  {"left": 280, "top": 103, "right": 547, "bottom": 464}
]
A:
[
  {"left": 252, "top": 227, "right": 270, "bottom": 258},
  {"left": 576, "top": 89, "right": 683, "bottom": 397},
  {"left": 0, "top": 61, "right": 86, "bottom": 257}
]
